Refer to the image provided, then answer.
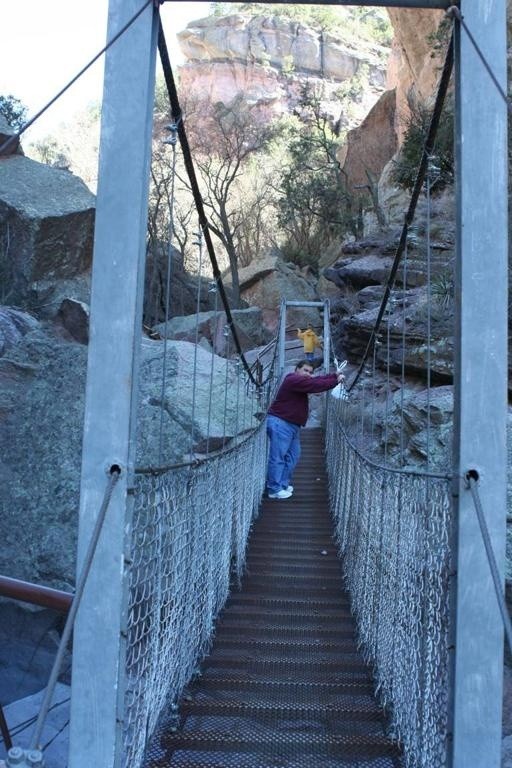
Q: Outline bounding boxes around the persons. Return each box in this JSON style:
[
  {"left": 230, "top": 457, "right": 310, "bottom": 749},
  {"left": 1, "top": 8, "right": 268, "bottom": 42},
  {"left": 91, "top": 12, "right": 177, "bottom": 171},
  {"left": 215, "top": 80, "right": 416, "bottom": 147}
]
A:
[
  {"left": 296, "top": 324, "right": 323, "bottom": 360},
  {"left": 263, "top": 359, "right": 345, "bottom": 499}
]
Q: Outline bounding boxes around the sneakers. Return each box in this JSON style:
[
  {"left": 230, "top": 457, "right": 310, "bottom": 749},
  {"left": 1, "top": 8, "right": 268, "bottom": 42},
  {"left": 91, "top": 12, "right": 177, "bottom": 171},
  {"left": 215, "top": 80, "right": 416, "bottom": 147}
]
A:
[
  {"left": 285, "top": 485, "right": 293, "bottom": 492},
  {"left": 268, "top": 490, "right": 293, "bottom": 498}
]
